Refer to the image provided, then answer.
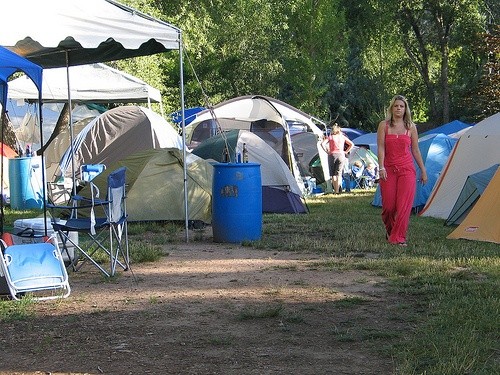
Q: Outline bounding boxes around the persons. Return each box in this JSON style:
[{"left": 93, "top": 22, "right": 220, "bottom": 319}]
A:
[
  {"left": 321, "top": 123, "right": 354, "bottom": 194},
  {"left": 376, "top": 94, "right": 427, "bottom": 247}
]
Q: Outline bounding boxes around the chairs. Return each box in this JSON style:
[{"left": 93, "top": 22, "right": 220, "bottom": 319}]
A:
[
  {"left": 0, "top": 164, "right": 133, "bottom": 300},
  {"left": 352, "top": 164, "right": 376, "bottom": 190}
]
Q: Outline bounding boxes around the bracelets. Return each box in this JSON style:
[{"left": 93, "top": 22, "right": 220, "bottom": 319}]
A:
[{"left": 379, "top": 168, "right": 385, "bottom": 171}]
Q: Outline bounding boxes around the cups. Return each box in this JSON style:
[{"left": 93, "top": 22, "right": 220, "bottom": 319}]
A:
[{"left": 31, "top": 150, "right": 36, "bottom": 157}]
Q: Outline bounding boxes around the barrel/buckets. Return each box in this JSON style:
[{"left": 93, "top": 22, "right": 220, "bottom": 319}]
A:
[
  {"left": 211, "top": 162, "right": 263, "bottom": 243},
  {"left": 8, "top": 156, "right": 49, "bottom": 210}
]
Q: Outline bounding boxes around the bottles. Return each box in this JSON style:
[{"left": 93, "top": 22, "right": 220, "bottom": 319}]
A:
[
  {"left": 18, "top": 145, "right": 24, "bottom": 157},
  {"left": 26, "top": 145, "right": 31, "bottom": 157},
  {"left": 242, "top": 143, "right": 249, "bottom": 163}
]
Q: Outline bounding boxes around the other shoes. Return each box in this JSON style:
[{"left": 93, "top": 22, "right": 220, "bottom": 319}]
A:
[
  {"left": 386, "top": 232, "right": 390, "bottom": 240},
  {"left": 339, "top": 187, "right": 342, "bottom": 193},
  {"left": 399, "top": 242, "right": 408, "bottom": 247}
]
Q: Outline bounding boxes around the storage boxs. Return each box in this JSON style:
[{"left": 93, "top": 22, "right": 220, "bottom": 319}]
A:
[{"left": 14, "top": 218, "right": 79, "bottom": 265}]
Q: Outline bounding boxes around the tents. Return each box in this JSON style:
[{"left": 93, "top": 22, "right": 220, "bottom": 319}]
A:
[
  {"left": 0, "top": 0, "right": 380, "bottom": 244},
  {"left": 371, "top": 113, "right": 500, "bottom": 244}
]
